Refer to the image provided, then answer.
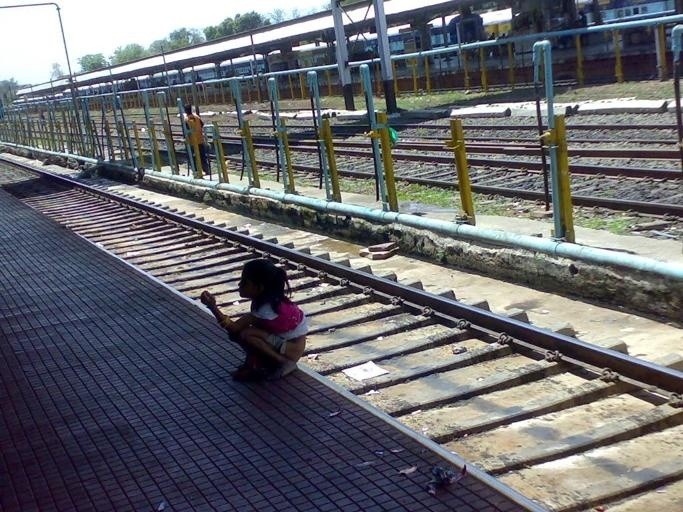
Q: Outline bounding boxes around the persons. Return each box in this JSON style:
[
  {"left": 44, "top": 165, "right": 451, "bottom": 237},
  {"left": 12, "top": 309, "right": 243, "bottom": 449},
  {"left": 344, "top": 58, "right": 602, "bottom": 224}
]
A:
[
  {"left": 183, "top": 103, "right": 210, "bottom": 176},
  {"left": 199, "top": 259, "right": 307, "bottom": 382}
]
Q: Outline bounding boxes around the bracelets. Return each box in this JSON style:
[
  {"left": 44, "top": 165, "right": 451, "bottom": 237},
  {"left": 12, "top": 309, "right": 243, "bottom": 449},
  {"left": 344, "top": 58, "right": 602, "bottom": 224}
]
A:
[{"left": 218, "top": 314, "right": 230, "bottom": 329}]
